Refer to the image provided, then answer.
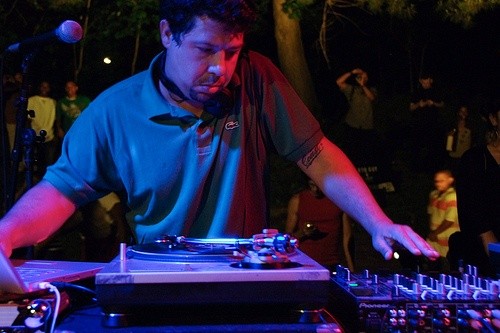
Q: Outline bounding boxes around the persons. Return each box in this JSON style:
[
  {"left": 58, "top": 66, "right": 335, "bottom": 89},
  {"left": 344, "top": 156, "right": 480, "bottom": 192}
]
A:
[
  {"left": 0, "top": 62, "right": 97, "bottom": 178},
  {"left": 284, "top": 65, "right": 500, "bottom": 275},
  {"left": 1, "top": 0, "right": 441, "bottom": 262}
]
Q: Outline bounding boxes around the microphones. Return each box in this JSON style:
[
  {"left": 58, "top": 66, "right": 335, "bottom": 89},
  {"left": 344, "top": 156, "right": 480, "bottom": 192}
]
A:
[{"left": 7, "top": 21, "right": 84, "bottom": 52}]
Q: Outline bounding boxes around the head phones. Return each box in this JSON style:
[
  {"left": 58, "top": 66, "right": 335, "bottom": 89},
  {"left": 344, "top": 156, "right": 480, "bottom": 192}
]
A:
[{"left": 159, "top": 48, "right": 236, "bottom": 119}]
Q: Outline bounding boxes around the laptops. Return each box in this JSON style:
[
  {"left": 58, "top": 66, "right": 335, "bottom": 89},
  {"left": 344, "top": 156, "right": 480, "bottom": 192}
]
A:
[{"left": 0, "top": 247, "right": 109, "bottom": 294}]
